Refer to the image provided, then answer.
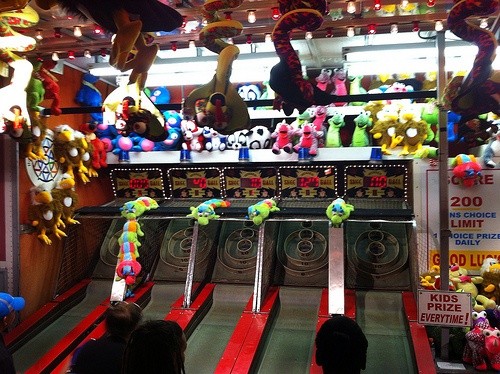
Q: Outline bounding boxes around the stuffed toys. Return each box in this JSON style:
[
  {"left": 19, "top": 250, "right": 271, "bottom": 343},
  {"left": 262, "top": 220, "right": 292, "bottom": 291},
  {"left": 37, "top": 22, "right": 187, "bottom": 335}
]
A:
[
  {"left": 0, "top": 0, "right": 500, "bottom": 284},
  {"left": 419, "top": 258, "right": 500, "bottom": 369}
]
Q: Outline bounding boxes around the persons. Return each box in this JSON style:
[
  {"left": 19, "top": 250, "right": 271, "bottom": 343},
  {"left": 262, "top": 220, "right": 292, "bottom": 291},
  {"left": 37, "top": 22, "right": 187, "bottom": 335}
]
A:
[
  {"left": 316, "top": 316, "right": 368, "bottom": 374},
  {"left": 71, "top": 301, "right": 187, "bottom": 374},
  {"left": 0, "top": 292, "right": 26, "bottom": 374}
]
